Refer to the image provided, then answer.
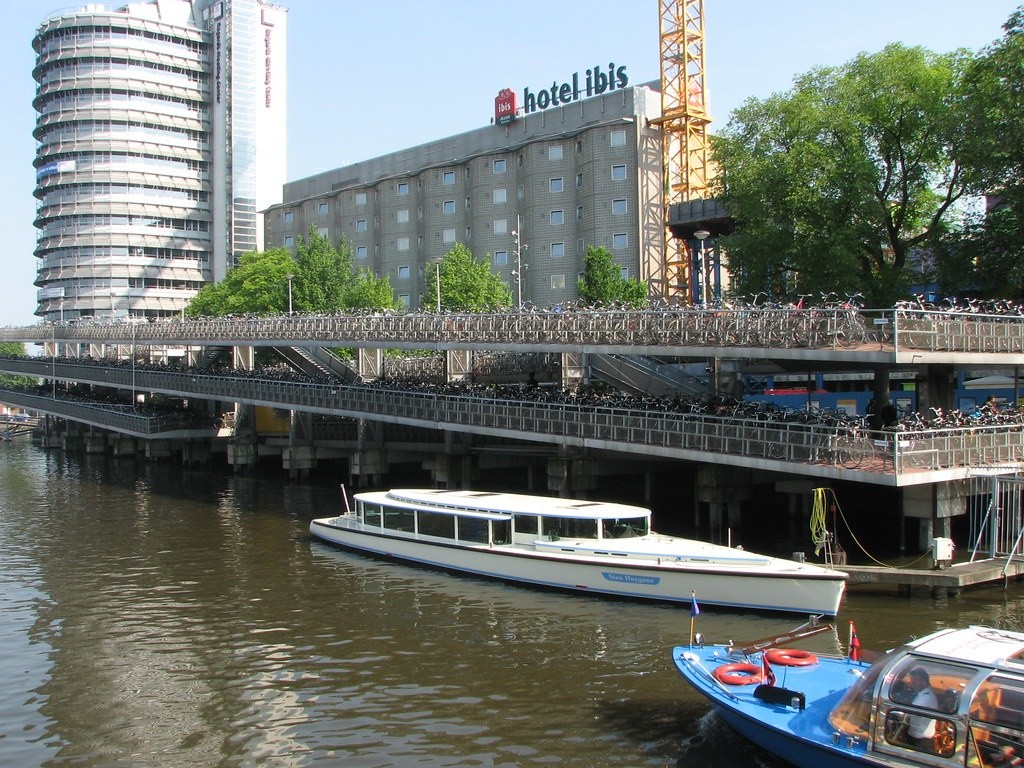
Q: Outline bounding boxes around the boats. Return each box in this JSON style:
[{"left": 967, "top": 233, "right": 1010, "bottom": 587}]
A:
[
  {"left": 671, "top": 588, "right": 1024, "bottom": 768},
  {"left": 309, "top": 481, "right": 852, "bottom": 618}
]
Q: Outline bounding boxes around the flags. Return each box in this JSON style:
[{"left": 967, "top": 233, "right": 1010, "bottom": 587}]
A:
[{"left": 850, "top": 625, "right": 859, "bottom": 660}]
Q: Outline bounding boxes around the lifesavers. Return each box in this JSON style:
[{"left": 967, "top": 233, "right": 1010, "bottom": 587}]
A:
[
  {"left": 713, "top": 662, "right": 766, "bottom": 685},
  {"left": 764, "top": 650, "right": 818, "bottom": 666}
]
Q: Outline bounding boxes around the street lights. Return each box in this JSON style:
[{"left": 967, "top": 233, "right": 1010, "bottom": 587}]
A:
[
  {"left": 286, "top": 273, "right": 296, "bottom": 318},
  {"left": 110, "top": 292, "right": 117, "bottom": 323},
  {"left": 434, "top": 257, "right": 445, "bottom": 313},
  {"left": 180, "top": 284, "right": 186, "bottom": 323},
  {"left": 694, "top": 230, "right": 710, "bottom": 308},
  {"left": 58, "top": 297, "right": 66, "bottom": 324}
]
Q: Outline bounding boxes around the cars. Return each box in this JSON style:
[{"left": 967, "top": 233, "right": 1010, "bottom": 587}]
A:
[{"left": 13, "top": 413, "right": 31, "bottom": 423}]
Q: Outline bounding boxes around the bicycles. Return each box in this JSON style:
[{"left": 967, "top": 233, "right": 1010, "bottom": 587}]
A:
[{"left": 0, "top": 289, "right": 1024, "bottom": 471}]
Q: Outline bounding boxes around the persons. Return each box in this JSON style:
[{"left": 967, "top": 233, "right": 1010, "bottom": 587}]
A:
[
  {"left": 984, "top": 395, "right": 997, "bottom": 411},
  {"left": 991, "top": 739, "right": 1024, "bottom": 768},
  {"left": 896, "top": 668, "right": 939, "bottom": 753},
  {"left": 865, "top": 398, "right": 896, "bottom": 439}
]
